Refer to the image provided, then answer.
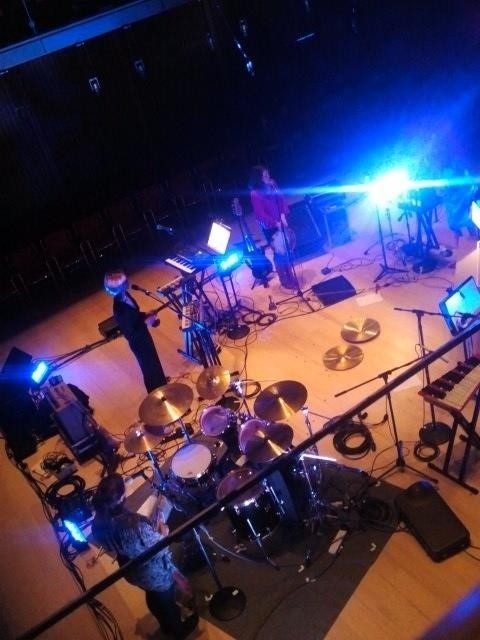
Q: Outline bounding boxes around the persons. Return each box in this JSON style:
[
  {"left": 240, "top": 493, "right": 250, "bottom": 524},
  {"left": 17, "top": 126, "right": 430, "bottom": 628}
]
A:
[
  {"left": 91, "top": 473, "right": 199, "bottom": 640},
  {"left": 249, "top": 165, "right": 299, "bottom": 290},
  {"left": 436, "top": 149, "right": 478, "bottom": 237},
  {"left": 104, "top": 272, "right": 167, "bottom": 396}
]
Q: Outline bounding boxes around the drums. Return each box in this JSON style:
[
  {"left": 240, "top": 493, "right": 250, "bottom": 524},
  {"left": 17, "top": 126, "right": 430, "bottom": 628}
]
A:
[
  {"left": 238, "top": 418, "right": 271, "bottom": 454},
  {"left": 199, "top": 406, "right": 236, "bottom": 437},
  {"left": 218, "top": 467, "right": 286, "bottom": 537},
  {"left": 170, "top": 445, "right": 216, "bottom": 487}
]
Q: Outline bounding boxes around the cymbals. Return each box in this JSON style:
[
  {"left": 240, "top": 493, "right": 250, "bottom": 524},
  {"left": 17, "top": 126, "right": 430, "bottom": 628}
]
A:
[
  {"left": 254, "top": 381, "right": 306, "bottom": 420},
  {"left": 125, "top": 422, "right": 164, "bottom": 454},
  {"left": 197, "top": 367, "right": 232, "bottom": 401},
  {"left": 139, "top": 384, "right": 192, "bottom": 426},
  {"left": 245, "top": 423, "right": 293, "bottom": 464},
  {"left": 342, "top": 318, "right": 381, "bottom": 343},
  {"left": 324, "top": 345, "right": 362, "bottom": 370}
]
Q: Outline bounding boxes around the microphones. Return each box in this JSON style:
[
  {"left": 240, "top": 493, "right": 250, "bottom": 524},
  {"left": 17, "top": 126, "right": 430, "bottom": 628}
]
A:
[
  {"left": 418, "top": 344, "right": 448, "bottom": 363},
  {"left": 456, "top": 310, "right": 480, "bottom": 321},
  {"left": 131, "top": 283, "right": 146, "bottom": 294}
]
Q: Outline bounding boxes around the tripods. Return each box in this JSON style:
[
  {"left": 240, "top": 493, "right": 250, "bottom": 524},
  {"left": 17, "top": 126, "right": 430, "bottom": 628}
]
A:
[
  {"left": 373, "top": 220, "right": 408, "bottom": 282},
  {"left": 375, "top": 379, "right": 438, "bottom": 483},
  {"left": 222, "top": 275, "right": 262, "bottom": 324},
  {"left": 235, "top": 207, "right": 277, "bottom": 294},
  {"left": 275, "top": 237, "right": 315, "bottom": 312},
  {"left": 237, "top": 483, "right": 352, "bottom": 574}
]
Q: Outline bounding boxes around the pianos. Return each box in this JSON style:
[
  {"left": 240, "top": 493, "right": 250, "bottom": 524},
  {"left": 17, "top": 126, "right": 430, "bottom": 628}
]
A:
[{"left": 165, "top": 244, "right": 214, "bottom": 279}]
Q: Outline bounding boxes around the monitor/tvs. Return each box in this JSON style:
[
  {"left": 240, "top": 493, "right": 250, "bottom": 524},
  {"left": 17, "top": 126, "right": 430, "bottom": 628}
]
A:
[
  {"left": 439, "top": 276, "right": 480, "bottom": 336},
  {"left": 203, "top": 220, "right": 232, "bottom": 256}
]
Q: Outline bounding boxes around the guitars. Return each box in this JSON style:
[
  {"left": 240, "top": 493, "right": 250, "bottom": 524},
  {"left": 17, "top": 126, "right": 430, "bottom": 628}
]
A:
[{"left": 232, "top": 195, "right": 272, "bottom": 278}]
